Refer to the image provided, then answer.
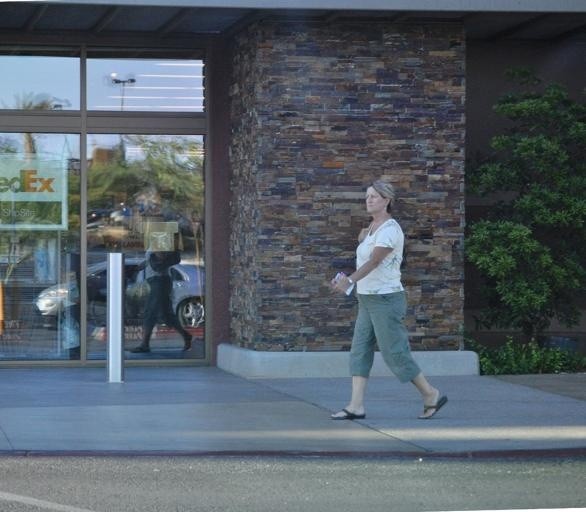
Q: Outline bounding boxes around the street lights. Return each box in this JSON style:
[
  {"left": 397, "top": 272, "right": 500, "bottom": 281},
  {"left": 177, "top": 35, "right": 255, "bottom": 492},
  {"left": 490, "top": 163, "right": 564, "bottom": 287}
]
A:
[{"left": 109, "top": 71, "right": 137, "bottom": 111}]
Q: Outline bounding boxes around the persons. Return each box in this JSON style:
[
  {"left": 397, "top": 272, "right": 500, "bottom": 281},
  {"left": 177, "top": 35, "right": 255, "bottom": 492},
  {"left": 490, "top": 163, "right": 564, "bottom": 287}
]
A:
[
  {"left": 116, "top": 213, "right": 193, "bottom": 354},
  {"left": 327, "top": 178, "right": 450, "bottom": 422}
]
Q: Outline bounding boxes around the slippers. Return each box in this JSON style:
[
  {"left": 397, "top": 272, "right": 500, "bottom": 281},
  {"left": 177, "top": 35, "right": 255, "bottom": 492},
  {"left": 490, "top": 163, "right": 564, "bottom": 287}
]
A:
[
  {"left": 183, "top": 335, "right": 191, "bottom": 351},
  {"left": 129, "top": 347, "right": 150, "bottom": 353},
  {"left": 417, "top": 395, "right": 448, "bottom": 420},
  {"left": 328, "top": 409, "right": 366, "bottom": 420}
]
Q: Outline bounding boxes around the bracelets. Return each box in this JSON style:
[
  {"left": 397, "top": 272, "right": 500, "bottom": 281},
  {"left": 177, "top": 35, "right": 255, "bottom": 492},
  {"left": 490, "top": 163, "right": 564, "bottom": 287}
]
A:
[{"left": 347, "top": 277, "right": 356, "bottom": 287}]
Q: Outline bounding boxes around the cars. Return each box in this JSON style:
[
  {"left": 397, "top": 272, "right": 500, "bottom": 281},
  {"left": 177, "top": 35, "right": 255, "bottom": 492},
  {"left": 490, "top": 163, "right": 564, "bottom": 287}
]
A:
[{"left": 31, "top": 256, "right": 206, "bottom": 334}]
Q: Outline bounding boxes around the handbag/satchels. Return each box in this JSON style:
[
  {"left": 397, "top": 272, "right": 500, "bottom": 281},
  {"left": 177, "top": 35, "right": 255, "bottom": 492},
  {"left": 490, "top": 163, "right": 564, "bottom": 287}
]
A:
[{"left": 149, "top": 247, "right": 183, "bottom": 273}]
